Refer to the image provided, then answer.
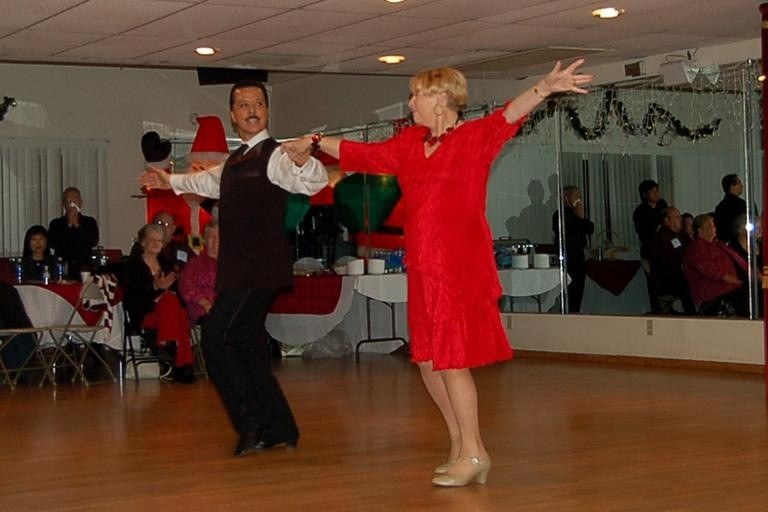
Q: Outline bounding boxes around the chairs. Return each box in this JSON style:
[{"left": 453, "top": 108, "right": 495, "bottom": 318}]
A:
[{"left": 0, "top": 249, "right": 206, "bottom": 397}]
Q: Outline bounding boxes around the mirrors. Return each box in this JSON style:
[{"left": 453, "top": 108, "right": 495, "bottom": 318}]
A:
[{"left": 461, "top": 59, "right": 768, "bottom": 325}]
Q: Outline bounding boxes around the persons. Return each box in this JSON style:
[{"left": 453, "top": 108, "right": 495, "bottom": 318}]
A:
[
  {"left": 23, "top": 187, "right": 220, "bottom": 385},
  {"left": 633, "top": 172, "right": 765, "bottom": 320},
  {"left": 278, "top": 54, "right": 595, "bottom": 485},
  {"left": 139, "top": 81, "right": 327, "bottom": 455},
  {"left": 551, "top": 185, "right": 594, "bottom": 313}
]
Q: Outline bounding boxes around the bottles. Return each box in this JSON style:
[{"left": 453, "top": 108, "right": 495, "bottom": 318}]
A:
[
  {"left": 373, "top": 249, "right": 405, "bottom": 274},
  {"left": 56, "top": 256, "right": 66, "bottom": 284},
  {"left": 15, "top": 261, "right": 23, "bottom": 284},
  {"left": 42, "top": 264, "right": 51, "bottom": 285}
]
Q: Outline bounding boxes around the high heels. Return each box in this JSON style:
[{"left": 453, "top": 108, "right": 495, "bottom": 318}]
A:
[{"left": 431, "top": 452, "right": 492, "bottom": 487}]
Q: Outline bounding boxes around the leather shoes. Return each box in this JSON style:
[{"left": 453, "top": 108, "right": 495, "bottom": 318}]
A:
[
  {"left": 253, "top": 430, "right": 299, "bottom": 450},
  {"left": 234, "top": 420, "right": 273, "bottom": 457},
  {"left": 156, "top": 342, "right": 197, "bottom": 384}
]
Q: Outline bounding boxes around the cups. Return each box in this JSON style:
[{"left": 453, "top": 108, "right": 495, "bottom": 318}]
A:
[
  {"left": 158, "top": 269, "right": 169, "bottom": 280},
  {"left": 511, "top": 255, "right": 528, "bottom": 269},
  {"left": 534, "top": 254, "right": 550, "bottom": 268},
  {"left": 367, "top": 259, "right": 385, "bottom": 275},
  {"left": 347, "top": 258, "right": 365, "bottom": 276},
  {"left": 79, "top": 271, "right": 90, "bottom": 283}
]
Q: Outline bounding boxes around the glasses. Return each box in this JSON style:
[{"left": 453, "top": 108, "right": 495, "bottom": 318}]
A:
[{"left": 155, "top": 220, "right": 174, "bottom": 227}]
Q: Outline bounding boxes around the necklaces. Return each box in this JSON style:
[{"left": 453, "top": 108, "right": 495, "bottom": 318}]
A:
[{"left": 423, "top": 117, "right": 461, "bottom": 147}]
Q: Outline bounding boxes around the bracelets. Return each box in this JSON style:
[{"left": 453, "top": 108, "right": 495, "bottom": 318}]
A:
[
  {"left": 533, "top": 84, "right": 548, "bottom": 100},
  {"left": 311, "top": 131, "right": 323, "bottom": 148}
]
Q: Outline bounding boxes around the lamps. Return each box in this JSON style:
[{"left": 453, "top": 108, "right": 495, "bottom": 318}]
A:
[
  {"left": 690, "top": 64, "right": 720, "bottom": 87},
  {"left": 655, "top": 47, "right": 702, "bottom": 88}
]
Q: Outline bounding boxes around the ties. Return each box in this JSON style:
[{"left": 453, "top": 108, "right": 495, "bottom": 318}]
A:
[{"left": 235, "top": 144, "right": 249, "bottom": 164}]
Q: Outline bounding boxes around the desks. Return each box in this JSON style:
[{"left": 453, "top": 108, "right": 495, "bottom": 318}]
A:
[
  {"left": 581, "top": 257, "right": 652, "bottom": 315},
  {"left": 261, "top": 272, "right": 408, "bottom": 365},
  {"left": 496, "top": 266, "right": 571, "bottom": 314}
]
